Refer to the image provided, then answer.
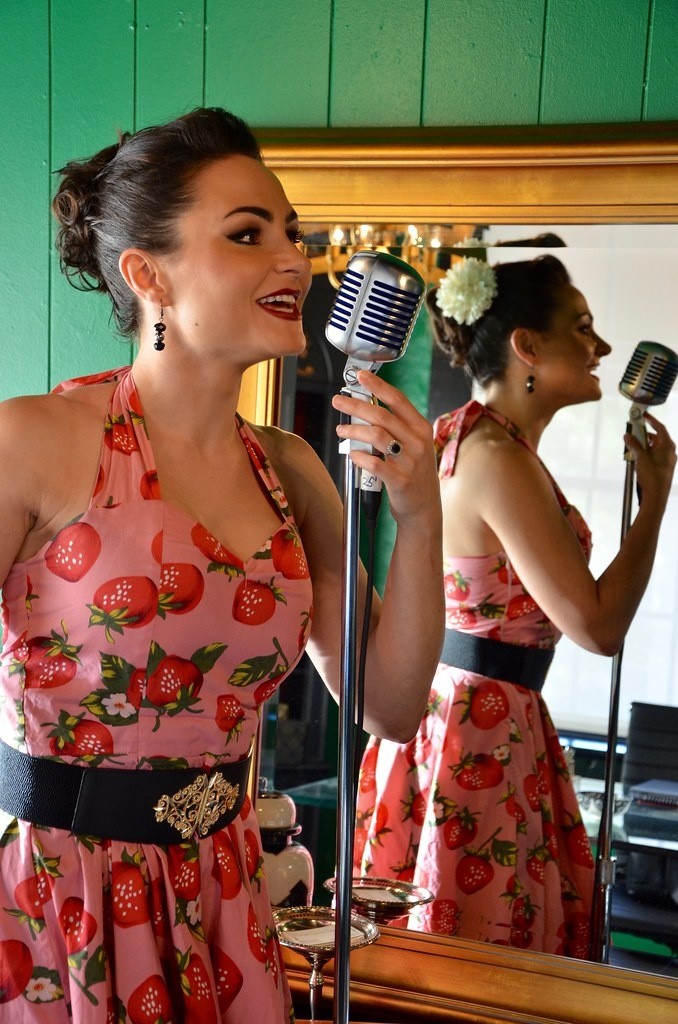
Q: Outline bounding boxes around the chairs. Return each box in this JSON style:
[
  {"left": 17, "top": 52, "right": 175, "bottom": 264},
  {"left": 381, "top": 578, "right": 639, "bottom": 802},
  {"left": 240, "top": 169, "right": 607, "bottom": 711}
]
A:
[{"left": 609, "top": 701, "right": 678, "bottom": 955}]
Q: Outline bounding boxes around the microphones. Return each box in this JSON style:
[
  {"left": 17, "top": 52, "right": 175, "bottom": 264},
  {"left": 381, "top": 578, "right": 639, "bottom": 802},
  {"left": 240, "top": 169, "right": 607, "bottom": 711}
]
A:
[
  {"left": 618, "top": 339, "right": 678, "bottom": 504},
  {"left": 325, "top": 248, "right": 425, "bottom": 522}
]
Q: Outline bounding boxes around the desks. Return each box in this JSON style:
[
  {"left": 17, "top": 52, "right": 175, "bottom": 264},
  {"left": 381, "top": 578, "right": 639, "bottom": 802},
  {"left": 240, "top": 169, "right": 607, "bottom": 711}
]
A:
[{"left": 562, "top": 771, "right": 678, "bottom": 849}]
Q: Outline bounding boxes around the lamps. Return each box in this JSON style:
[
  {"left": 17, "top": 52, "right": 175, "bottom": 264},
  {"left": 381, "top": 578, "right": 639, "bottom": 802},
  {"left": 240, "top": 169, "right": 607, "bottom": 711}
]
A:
[{"left": 300, "top": 218, "right": 487, "bottom": 293}]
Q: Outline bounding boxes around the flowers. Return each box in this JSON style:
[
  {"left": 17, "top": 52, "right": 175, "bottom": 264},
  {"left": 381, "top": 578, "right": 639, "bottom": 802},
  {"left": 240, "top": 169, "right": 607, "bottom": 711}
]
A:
[{"left": 433, "top": 254, "right": 500, "bottom": 326}]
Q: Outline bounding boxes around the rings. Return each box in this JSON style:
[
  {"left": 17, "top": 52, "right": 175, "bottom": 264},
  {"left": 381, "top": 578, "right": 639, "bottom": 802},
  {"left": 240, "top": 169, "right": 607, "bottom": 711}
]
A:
[{"left": 385, "top": 440, "right": 403, "bottom": 456}]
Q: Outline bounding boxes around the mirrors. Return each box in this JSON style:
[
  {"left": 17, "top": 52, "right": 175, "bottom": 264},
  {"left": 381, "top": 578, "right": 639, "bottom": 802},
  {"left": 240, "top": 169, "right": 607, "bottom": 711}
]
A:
[{"left": 238, "top": 122, "right": 678, "bottom": 1024}]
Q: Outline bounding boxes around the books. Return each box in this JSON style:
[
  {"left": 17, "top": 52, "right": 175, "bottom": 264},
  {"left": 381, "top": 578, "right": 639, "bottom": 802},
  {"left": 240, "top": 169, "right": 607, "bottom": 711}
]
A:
[{"left": 628, "top": 777, "right": 678, "bottom": 809}]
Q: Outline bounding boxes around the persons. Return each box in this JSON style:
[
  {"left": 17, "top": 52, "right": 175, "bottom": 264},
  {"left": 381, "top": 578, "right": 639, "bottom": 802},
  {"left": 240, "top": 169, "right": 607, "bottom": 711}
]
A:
[
  {"left": 331, "top": 253, "right": 678, "bottom": 957},
  {"left": 0, "top": 103, "right": 443, "bottom": 1024}
]
